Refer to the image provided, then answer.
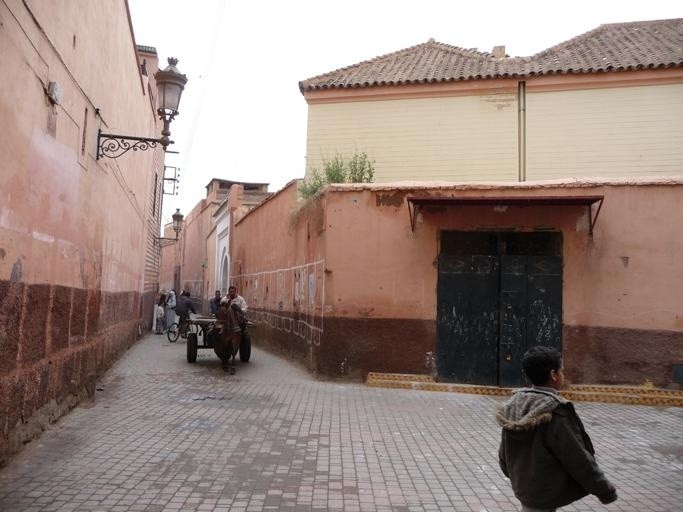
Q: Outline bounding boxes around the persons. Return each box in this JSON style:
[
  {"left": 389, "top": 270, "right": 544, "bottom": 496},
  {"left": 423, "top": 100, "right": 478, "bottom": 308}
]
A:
[
  {"left": 219, "top": 285, "right": 247, "bottom": 313},
  {"left": 209, "top": 290, "right": 221, "bottom": 314},
  {"left": 496, "top": 345, "right": 617, "bottom": 511},
  {"left": 175, "top": 292, "right": 196, "bottom": 337},
  {"left": 153, "top": 287, "right": 176, "bottom": 335}
]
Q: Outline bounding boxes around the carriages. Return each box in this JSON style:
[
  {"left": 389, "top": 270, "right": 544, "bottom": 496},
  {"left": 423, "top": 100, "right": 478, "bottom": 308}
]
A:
[{"left": 184, "top": 298, "right": 256, "bottom": 376}]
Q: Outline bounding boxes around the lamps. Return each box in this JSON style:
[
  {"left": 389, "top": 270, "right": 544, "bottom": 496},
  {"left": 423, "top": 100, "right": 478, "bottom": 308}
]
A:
[
  {"left": 92, "top": 52, "right": 189, "bottom": 165},
  {"left": 152, "top": 208, "right": 185, "bottom": 246}
]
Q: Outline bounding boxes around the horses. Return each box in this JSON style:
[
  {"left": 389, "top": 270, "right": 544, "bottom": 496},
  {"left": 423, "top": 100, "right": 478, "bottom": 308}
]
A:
[{"left": 213, "top": 298, "right": 242, "bottom": 375}]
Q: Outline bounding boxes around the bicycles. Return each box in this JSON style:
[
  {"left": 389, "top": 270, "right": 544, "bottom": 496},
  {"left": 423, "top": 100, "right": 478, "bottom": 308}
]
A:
[{"left": 165, "top": 310, "right": 198, "bottom": 342}]
